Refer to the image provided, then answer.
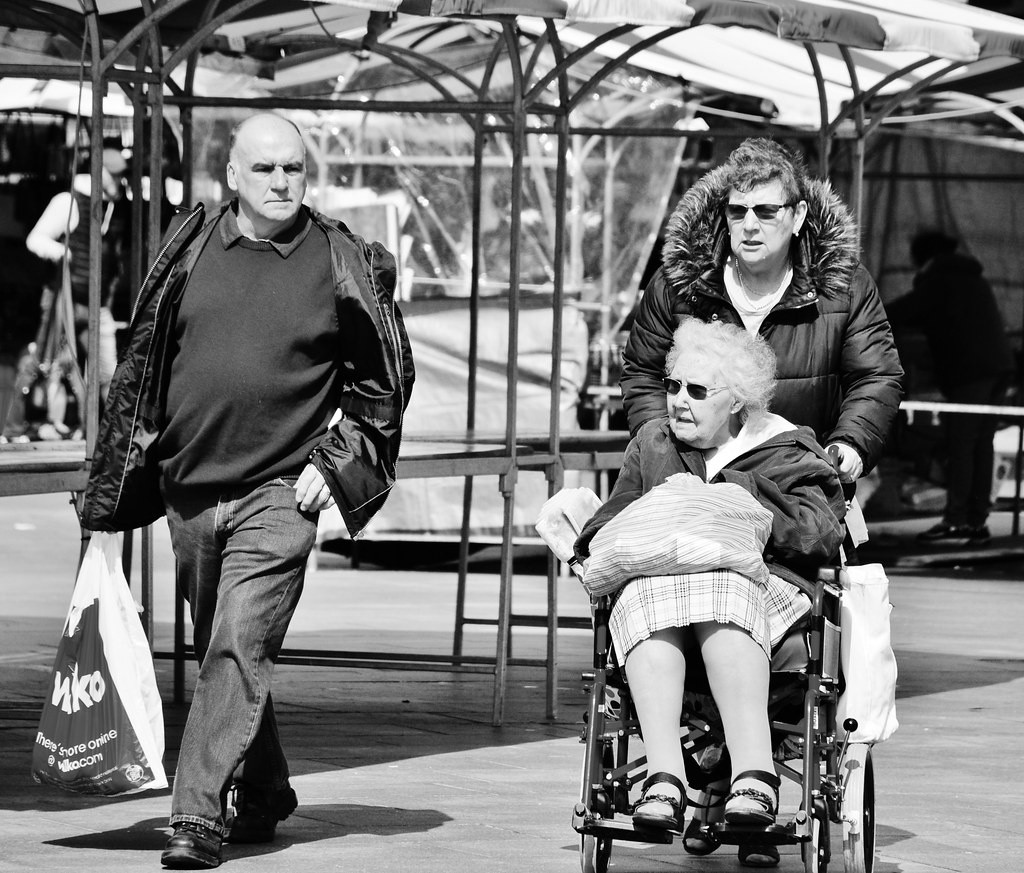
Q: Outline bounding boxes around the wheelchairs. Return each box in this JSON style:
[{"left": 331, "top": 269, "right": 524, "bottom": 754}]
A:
[{"left": 575, "top": 444, "right": 881, "bottom": 873}]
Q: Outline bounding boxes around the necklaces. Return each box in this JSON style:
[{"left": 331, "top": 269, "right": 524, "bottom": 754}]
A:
[{"left": 735, "top": 256, "right": 789, "bottom": 309}]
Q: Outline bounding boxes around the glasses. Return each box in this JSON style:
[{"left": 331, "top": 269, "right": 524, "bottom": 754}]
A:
[
  {"left": 722, "top": 198, "right": 797, "bottom": 220},
  {"left": 662, "top": 377, "right": 728, "bottom": 400}
]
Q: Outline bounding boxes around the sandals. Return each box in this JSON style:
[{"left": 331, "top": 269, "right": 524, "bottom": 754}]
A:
[
  {"left": 738, "top": 844, "right": 780, "bottom": 866},
  {"left": 722, "top": 770, "right": 782, "bottom": 826},
  {"left": 631, "top": 772, "right": 688, "bottom": 836},
  {"left": 682, "top": 783, "right": 731, "bottom": 855}
]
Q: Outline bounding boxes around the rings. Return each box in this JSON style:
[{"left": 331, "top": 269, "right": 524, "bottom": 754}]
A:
[{"left": 848, "top": 476, "right": 854, "bottom": 482}]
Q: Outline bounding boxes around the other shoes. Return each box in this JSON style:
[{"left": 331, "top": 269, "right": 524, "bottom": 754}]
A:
[{"left": 917, "top": 525, "right": 989, "bottom": 543}]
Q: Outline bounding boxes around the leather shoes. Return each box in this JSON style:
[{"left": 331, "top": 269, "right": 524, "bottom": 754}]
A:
[
  {"left": 225, "top": 787, "right": 298, "bottom": 842},
  {"left": 160, "top": 822, "right": 224, "bottom": 867}
]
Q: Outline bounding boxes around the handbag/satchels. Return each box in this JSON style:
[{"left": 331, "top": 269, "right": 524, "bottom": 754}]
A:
[{"left": 29, "top": 531, "right": 169, "bottom": 797}]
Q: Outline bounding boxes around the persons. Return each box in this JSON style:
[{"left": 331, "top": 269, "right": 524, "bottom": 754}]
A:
[
  {"left": 909, "top": 226, "right": 1010, "bottom": 544},
  {"left": 571, "top": 318, "right": 845, "bottom": 829},
  {"left": 621, "top": 137, "right": 905, "bottom": 868},
  {"left": 79, "top": 112, "right": 415, "bottom": 868},
  {"left": 0, "top": 157, "right": 125, "bottom": 444}
]
